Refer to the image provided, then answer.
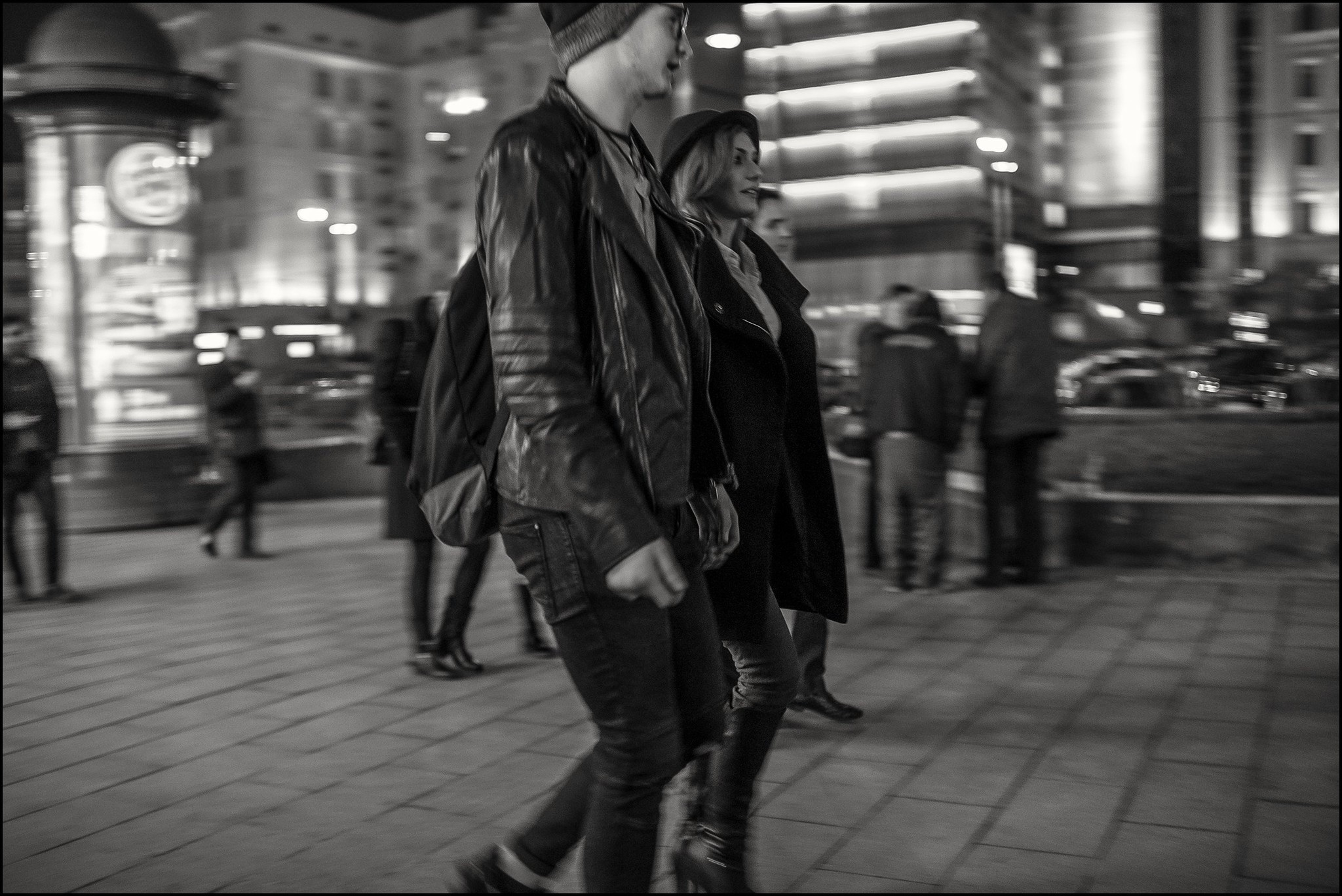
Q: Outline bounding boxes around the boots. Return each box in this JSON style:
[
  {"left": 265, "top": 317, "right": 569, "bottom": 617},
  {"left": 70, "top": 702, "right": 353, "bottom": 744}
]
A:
[
  {"left": 430, "top": 594, "right": 483, "bottom": 672},
  {"left": 670, "top": 696, "right": 710, "bottom": 893},
  {"left": 684, "top": 694, "right": 787, "bottom": 893},
  {"left": 408, "top": 599, "right": 461, "bottom": 679}
]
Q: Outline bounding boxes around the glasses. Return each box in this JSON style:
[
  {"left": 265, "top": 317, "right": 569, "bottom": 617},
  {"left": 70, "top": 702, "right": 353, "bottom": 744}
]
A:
[{"left": 653, "top": 2, "right": 690, "bottom": 40}]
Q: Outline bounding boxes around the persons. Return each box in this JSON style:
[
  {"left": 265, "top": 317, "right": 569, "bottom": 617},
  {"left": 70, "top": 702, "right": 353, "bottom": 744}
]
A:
[
  {"left": 848, "top": 285, "right": 918, "bottom": 577},
  {"left": 4, "top": 312, "right": 94, "bottom": 605},
  {"left": 855, "top": 290, "right": 970, "bottom": 597},
  {"left": 196, "top": 326, "right": 278, "bottom": 561},
  {"left": 659, "top": 108, "right": 848, "bottom": 894},
  {"left": 454, "top": 4, "right": 742, "bottom": 896},
  {"left": 966, "top": 271, "right": 1068, "bottom": 585},
  {"left": 796, "top": 603, "right": 869, "bottom": 722},
  {"left": 367, "top": 292, "right": 492, "bottom": 685}
]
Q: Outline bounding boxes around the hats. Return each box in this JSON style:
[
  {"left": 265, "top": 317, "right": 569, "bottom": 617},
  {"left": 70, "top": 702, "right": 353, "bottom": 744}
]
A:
[
  {"left": 658, "top": 108, "right": 760, "bottom": 184},
  {"left": 538, "top": 3, "right": 650, "bottom": 77}
]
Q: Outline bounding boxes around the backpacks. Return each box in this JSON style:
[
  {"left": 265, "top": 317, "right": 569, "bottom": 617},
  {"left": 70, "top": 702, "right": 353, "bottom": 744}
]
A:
[{"left": 403, "top": 247, "right": 513, "bottom": 550}]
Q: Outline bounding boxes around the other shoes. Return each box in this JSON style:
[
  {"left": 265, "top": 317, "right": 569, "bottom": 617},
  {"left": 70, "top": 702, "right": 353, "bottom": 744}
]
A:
[
  {"left": 13, "top": 588, "right": 37, "bottom": 602},
  {"left": 197, "top": 530, "right": 218, "bottom": 557},
  {"left": 861, "top": 560, "right": 1043, "bottom": 596},
  {"left": 47, "top": 586, "right": 79, "bottom": 602},
  {"left": 239, "top": 547, "right": 274, "bottom": 559}
]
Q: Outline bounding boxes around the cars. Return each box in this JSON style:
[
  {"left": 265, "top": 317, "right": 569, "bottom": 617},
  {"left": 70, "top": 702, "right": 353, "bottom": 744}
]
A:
[
  {"left": 1055, "top": 349, "right": 1185, "bottom": 409},
  {"left": 815, "top": 355, "right": 864, "bottom": 411},
  {"left": 1176, "top": 330, "right": 1342, "bottom": 407}
]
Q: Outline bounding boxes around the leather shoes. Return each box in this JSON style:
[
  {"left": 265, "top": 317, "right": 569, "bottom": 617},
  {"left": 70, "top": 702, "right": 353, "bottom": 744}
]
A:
[{"left": 788, "top": 676, "right": 865, "bottom": 722}]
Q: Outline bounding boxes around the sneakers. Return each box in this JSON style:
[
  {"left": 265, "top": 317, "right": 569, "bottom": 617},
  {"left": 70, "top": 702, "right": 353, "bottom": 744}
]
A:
[{"left": 442, "top": 841, "right": 550, "bottom": 893}]
